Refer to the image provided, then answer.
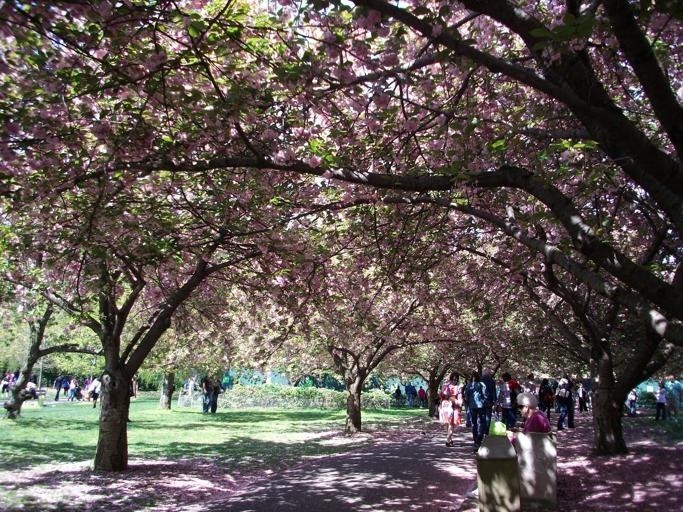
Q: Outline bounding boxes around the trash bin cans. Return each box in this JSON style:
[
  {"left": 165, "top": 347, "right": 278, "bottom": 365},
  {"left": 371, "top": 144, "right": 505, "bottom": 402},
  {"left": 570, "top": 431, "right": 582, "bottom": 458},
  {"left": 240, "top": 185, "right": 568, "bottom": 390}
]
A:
[{"left": 475, "top": 435, "right": 521, "bottom": 512}]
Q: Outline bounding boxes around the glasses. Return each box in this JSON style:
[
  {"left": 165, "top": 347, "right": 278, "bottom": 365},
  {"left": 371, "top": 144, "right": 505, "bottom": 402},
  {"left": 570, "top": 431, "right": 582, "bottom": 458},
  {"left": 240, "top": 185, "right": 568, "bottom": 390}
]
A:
[{"left": 518, "top": 405, "right": 523, "bottom": 409}]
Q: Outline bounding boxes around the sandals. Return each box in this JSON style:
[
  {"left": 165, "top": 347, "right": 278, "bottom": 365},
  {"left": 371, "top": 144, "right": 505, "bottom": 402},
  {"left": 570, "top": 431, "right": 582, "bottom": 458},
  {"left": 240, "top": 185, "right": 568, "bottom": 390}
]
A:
[{"left": 446, "top": 441, "right": 454, "bottom": 447}]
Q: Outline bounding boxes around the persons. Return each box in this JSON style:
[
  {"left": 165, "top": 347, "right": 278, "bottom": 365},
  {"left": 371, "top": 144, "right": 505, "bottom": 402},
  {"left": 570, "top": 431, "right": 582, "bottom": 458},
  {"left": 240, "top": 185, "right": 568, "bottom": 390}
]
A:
[
  {"left": 0, "top": 368, "right": 225, "bottom": 413},
  {"left": 465, "top": 391, "right": 552, "bottom": 502},
  {"left": 394, "top": 372, "right": 682, "bottom": 452}
]
